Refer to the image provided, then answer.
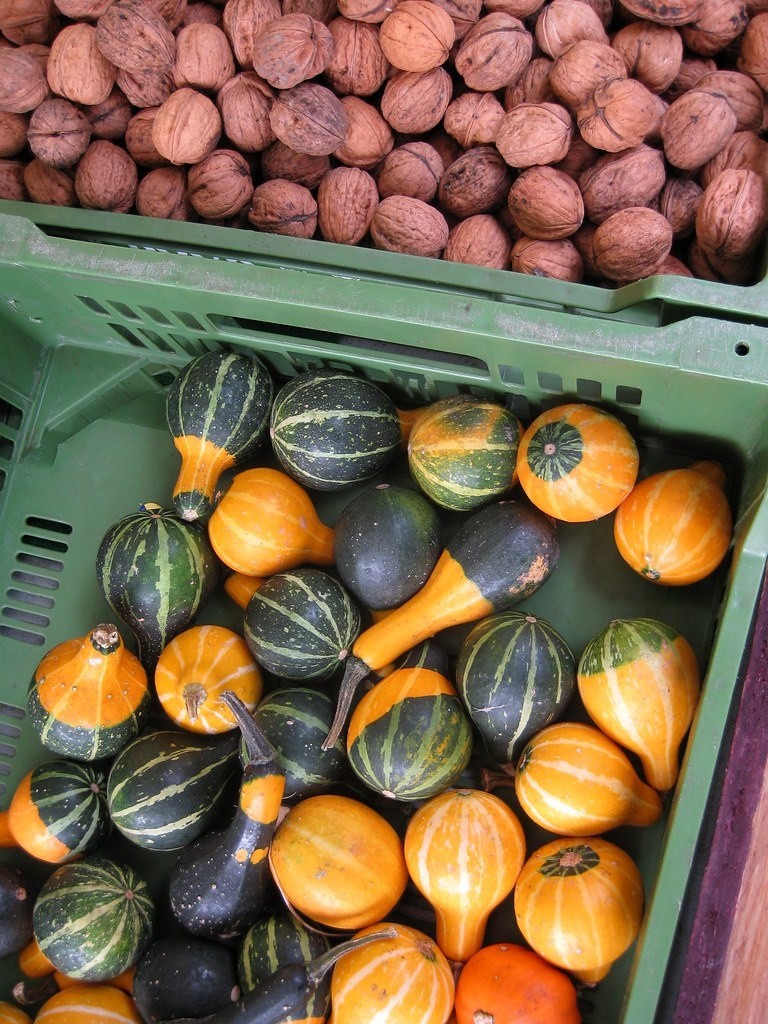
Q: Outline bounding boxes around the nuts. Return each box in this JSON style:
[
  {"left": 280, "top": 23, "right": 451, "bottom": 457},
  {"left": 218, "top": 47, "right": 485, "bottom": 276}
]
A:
[{"left": 1, "top": 0, "right": 768, "bottom": 287}]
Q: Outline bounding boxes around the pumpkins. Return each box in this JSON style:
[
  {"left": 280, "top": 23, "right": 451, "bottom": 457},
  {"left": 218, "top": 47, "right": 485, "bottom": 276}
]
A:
[{"left": 0, "top": 344, "right": 734, "bottom": 1024}]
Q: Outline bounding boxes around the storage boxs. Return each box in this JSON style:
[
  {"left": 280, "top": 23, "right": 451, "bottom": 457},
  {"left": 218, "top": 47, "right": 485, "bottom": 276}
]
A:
[{"left": 0, "top": 0, "right": 768, "bottom": 1024}]
